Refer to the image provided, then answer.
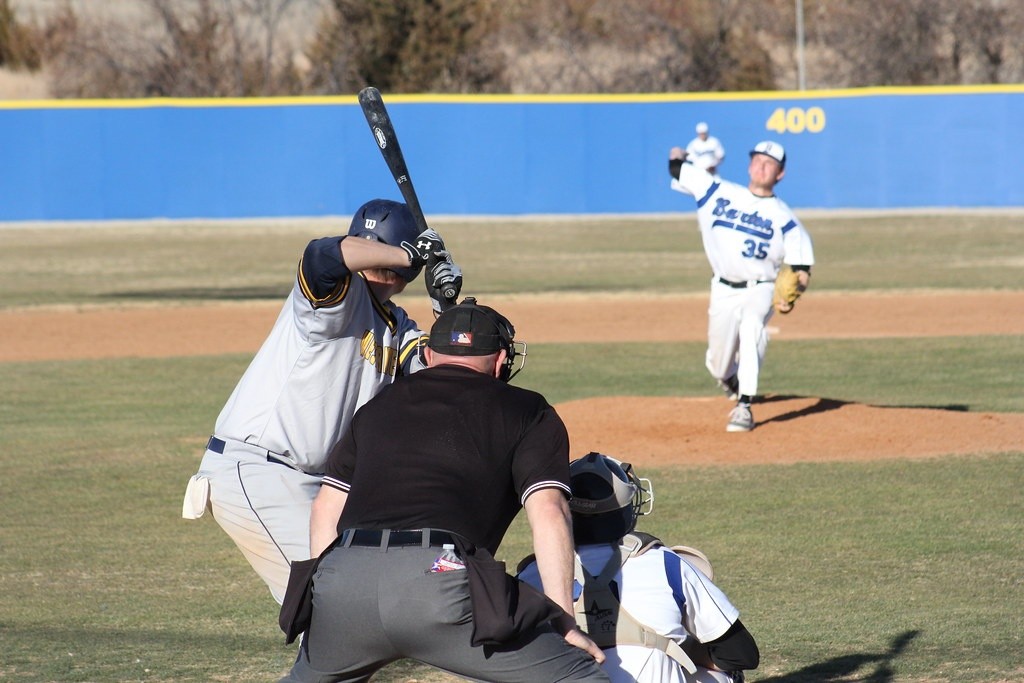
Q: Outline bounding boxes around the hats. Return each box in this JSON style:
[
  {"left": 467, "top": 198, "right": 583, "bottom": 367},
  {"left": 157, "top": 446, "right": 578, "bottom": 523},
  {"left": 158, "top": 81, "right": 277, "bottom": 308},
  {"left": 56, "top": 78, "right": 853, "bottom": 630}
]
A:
[
  {"left": 696, "top": 121, "right": 707, "bottom": 134},
  {"left": 429, "top": 304, "right": 504, "bottom": 356},
  {"left": 750, "top": 140, "right": 785, "bottom": 168}
]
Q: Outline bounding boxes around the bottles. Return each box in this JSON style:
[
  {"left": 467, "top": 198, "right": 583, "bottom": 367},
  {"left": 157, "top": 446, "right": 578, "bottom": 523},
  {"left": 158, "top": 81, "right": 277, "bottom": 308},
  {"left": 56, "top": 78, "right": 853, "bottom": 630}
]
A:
[{"left": 432, "top": 543, "right": 464, "bottom": 573}]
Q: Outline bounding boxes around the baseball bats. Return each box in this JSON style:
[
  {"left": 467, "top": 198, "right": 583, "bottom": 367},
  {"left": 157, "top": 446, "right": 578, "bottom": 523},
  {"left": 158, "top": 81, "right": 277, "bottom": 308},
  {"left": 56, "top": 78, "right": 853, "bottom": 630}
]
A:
[{"left": 356, "top": 85, "right": 459, "bottom": 299}]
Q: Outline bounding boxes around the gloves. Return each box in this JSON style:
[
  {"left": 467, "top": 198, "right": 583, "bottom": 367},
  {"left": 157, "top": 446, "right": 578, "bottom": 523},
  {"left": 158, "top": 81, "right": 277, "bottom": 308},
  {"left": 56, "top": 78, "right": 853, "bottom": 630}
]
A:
[
  {"left": 400, "top": 227, "right": 444, "bottom": 268},
  {"left": 425, "top": 243, "right": 462, "bottom": 318}
]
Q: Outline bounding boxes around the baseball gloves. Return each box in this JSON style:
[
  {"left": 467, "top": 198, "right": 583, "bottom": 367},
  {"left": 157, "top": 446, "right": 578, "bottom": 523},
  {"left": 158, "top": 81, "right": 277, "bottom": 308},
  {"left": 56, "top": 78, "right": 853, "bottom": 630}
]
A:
[{"left": 773, "top": 264, "right": 803, "bottom": 314}]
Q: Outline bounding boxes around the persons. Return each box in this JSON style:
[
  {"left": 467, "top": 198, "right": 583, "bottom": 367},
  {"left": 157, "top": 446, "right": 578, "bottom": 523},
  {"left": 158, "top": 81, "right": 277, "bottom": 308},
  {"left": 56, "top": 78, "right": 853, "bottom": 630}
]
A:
[
  {"left": 670, "top": 141, "right": 814, "bottom": 432},
  {"left": 516, "top": 452, "right": 760, "bottom": 683},
  {"left": 275, "top": 297, "right": 611, "bottom": 683},
  {"left": 686, "top": 123, "right": 726, "bottom": 174},
  {"left": 182, "top": 200, "right": 462, "bottom": 646}
]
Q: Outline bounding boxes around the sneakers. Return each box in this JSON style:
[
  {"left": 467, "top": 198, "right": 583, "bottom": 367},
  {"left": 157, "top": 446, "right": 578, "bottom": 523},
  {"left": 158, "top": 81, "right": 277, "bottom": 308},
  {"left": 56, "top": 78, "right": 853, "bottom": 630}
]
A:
[
  {"left": 726, "top": 402, "right": 754, "bottom": 432},
  {"left": 718, "top": 373, "right": 738, "bottom": 401}
]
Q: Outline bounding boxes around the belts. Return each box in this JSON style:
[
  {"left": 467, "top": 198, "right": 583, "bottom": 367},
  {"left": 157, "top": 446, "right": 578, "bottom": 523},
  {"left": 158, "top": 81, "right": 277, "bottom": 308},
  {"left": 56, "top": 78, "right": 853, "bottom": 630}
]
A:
[
  {"left": 338, "top": 529, "right": 453, "bottom": 547},
  {"left": 712, "top": 274, "right": 761, "bottom": 287},
  {"left": 207, "top": 434, "right": 293, "bottom": 468}
]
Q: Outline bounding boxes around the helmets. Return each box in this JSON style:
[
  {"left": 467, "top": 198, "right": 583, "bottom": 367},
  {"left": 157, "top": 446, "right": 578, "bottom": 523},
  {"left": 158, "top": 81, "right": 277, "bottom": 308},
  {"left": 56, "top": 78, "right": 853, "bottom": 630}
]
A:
[
  {"left": 349, "top": 198, "right": 424, "bottom": 281},
  {"left": 568, "top": 456, "right": 633, "bottom": 546}
]
